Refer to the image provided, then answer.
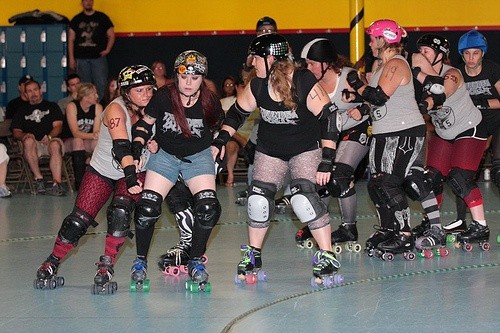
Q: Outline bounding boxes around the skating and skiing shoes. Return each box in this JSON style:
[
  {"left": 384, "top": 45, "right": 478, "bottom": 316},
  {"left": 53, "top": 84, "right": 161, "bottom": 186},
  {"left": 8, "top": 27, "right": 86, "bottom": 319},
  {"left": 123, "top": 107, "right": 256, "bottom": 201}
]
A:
[
  {"left": 34, "top": 254, "right": 65, "bottom": 289},
  {"left": 366, "top": 227, "right": 396, "bottom": 257},
  {"left": 159, "top": 244, "right": 209, "bottom": 276},
  {"left": 457, "top": 220, "right": 491, "bottom": 251},
  {"left": 442, "top": 219, "right": 468, "bottom": 243},
  {"left": 309, "top": 249, "right": 344, "bottom": 287},
  {"left": 330, "top": 221, "right": 362, "bottom": 254},
  {"left": 412, "top": 214, "right": 431, "bottom": 237},
  {"left": 296, "top": 225, "right": 318, "bottom": 248},
  {"left": 378, "top": 231, "right": 415, "bottom": 261},
  {"left": 236, "top": 187, "right": 251, "bottom": 205},
  {"left": 417, "top": 229, "right": 449, "bottom": 258},
  {"left": 185, "top": 256, "right": 213, "bottom": 293},
  {"left": 275, "top": 195, "right": 298, "bottom": 215},
  {"left": 235, "top": 245, "right": 267, "bottom": 286},
  {"left": 89, "top": 256, "right": 118, "bottom": 295},
  {"left": 129, "top": 257, "right": 150, "bottom": 293}
]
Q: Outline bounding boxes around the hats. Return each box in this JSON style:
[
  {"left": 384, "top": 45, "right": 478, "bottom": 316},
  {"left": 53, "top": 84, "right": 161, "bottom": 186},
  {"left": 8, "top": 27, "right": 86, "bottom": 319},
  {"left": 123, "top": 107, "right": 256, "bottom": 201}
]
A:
[
  {"left": 20, "top": 75, "right": 33, "bottom": 84},
  {"left": 366, "top": 19, "right": 405, "bottom": 45},
  {"left": 458, "top": 29, "right": 488, "bottom": 55},
  {"left": 118, "top": 64, "right": 157, "bottom": 93},
  {"left": 174, "top": 49, "right": 209, "bottom": 77},
  {"left": 256, "top": 15, "right": 276, "bottom": 28},
  {"left": 250, "top": 32, "right": 291, "bottom": 60},
  {"left": 300, "top": 37, "right": 337, "bottom": 63},
  {"left": 416, "top": 33, "right": 449, "bottom": 61}
]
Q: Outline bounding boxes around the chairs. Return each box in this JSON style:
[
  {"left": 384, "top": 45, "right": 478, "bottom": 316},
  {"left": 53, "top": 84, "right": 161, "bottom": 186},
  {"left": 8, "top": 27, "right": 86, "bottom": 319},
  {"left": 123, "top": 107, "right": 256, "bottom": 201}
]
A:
[{"left": 0, "top": 118, "right": 32, "bottom": 195}]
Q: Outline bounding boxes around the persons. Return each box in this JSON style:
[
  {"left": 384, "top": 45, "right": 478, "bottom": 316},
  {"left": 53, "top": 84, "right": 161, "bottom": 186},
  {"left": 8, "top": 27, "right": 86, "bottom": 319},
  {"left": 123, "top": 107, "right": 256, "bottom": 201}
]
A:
[
  {"left": 340, "top": 17, "right": 428, "bottom": 253},
  {"left": 34, "top": 62, "right": 160, "bottom": 287},
  {"left": 217, "top": 75, "right": 238, "bottom": 113},
  {"left": 6, "top": 75, "right": 37, "bottom": 154},
  {"left": 162, "top": 173, "right": 203, "bottom": 267},
  {"left": 130, "top": 49, "right": 226, "bottom": 283},
  {"left": 57, "top": 74, "right": 83, "bottom": 113},
  {"left": 291, "top": 34, "right": 372, "bottom": 245},
  {"left": 370, "top": 28, "right": 448, "bottom": 251},
  {"left": 107, "top": 78, "right": 119, "bottom": 103},
  {"left": 148, "top": 60, "right": 170, "bottom": 89},
  {"left": 59, "top": 82, "right": 104, "bottom": 192},
  {"left": 443, "top": 29, "right": 500, "bottom": 233},
  {"left": 234, "top": 11, "right": 292, "bottom": 206},
  {"left": 11, "top": 76, "right": 67, "bottom": 197},
  {"left": 1, "top": 142, "right": 13, "bottom": 198},
  {"left": 67, "top": 0, "right": 115, "bottom": 103},
  {"left": 410, "top": 32, "right": 492, "bottom": 243},
  {"left": 209, "top": 33, "right": 344, "bottom": 278}
]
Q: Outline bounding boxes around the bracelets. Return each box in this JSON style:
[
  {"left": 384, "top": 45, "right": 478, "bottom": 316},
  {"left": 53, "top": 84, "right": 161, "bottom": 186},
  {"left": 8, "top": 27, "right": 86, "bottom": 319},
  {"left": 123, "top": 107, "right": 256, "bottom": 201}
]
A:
[{"left": 47, "top": 134, "right": 52, "bottom": 142}]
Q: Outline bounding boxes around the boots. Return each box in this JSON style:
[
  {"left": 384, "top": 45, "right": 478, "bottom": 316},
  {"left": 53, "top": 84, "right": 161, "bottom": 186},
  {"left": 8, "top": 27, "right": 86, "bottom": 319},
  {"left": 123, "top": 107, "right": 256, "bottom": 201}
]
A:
[{"left": 71, "top": 149, "right": 86, "bottom": 192}]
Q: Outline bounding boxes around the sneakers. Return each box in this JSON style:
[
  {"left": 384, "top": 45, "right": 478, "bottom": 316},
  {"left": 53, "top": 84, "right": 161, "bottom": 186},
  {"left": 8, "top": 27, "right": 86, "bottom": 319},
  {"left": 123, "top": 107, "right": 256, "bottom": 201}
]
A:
[
  {"left": 0, "top": 186, "right": 12, "bottom": 198},
  {"left": 35, "top": 175, "right": 46, "bottom": 193},
  {"left": 53, "top": 183, "right": 66, "bottom": 197}
]
